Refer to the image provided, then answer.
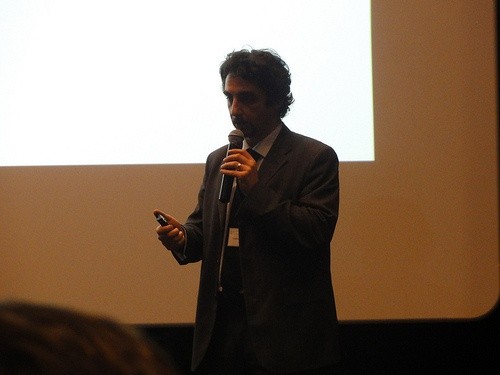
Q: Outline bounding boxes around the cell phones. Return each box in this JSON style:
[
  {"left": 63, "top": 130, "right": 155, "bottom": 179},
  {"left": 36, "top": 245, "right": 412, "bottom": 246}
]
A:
[{"left": 156, "top": 213, "right": 168, "bottom": 226}]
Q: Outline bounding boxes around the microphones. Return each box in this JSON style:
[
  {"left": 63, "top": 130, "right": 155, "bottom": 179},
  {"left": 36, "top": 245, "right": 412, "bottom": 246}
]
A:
[{"left": 218, "top": 130, "right": 244, "bottom": 204}]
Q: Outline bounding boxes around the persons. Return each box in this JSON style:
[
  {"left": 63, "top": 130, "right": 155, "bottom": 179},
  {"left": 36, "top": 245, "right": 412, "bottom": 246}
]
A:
[
  {"left": 0, "top": 299, "right": 171, "bottom": 374},
  {"left": 154, "top": 48, "right": 340, "bottom": 374}
]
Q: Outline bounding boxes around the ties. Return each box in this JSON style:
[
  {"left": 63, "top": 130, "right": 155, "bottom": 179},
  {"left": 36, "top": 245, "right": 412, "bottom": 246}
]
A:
[{"left": 223, "top": 150, "right": 259, "bottom": 293}]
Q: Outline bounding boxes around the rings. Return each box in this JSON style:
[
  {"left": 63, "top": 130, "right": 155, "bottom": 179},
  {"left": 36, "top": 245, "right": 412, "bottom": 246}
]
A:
[{"left": 235, "top": 163, "right": 241, "bottom": 170}]
